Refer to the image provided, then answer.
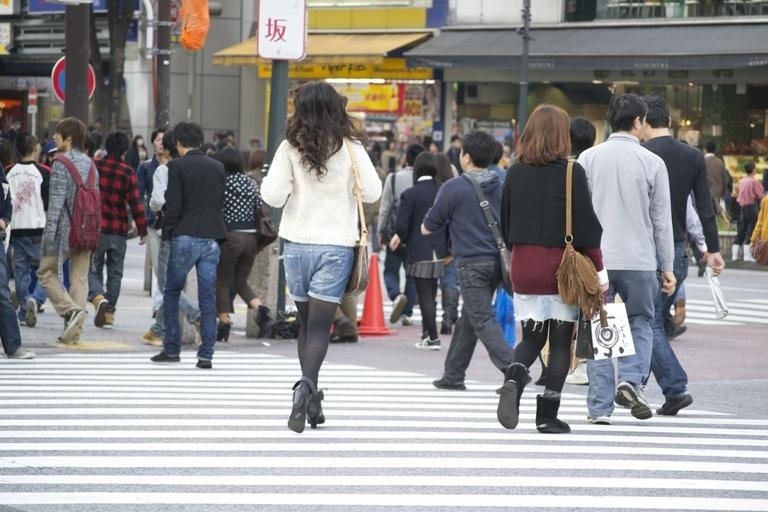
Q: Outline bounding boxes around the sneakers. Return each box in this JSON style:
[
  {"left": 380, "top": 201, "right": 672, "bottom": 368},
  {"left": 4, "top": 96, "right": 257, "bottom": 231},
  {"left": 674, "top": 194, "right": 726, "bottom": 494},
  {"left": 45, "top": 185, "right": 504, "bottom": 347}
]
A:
[
  {"left": 388, "top": 292, "right": 408, "bottom": 325},
  {"left": 149, "top": 351, "right": 181, "bottom": 364},
  {"left": 667, "top": 324, "right": 688, "bottom": 344},
  {"left": 54, "top": 326, "right": 84, "bottom": 344},
  {"left": 61, "top": 307, "right": 90, "bottom": 340},
  {"left": 401, "top": 316, "right": 415, "bottom": 325},
  {"left": 18, "top": 296, "right": 44, "bottom": 329},
  {"left": 413, "top": 320, "right": 454, "bottom": 351},
  {"left": 613, "top": 377, "right": 653, "bottom": 421},
  {"left": 656, "top": 393, "right": 696, "bottom": 419},
  {"left": 494, "top": 384, "right": 504, "bottom": 394},
  {"left": 5, "top": 347, "right": 38, "bottom": 360},
  {"left": 584, "top": 414, "right": 614, "bottom": 426},
  {"left": 431, "top": 376, "right": 467, "bottom": 391},
  {"left": 139, "top": 329, "right": 164, "bottom": 345},
  {"left": 195, "top": 359, "right": 213, "bottom": 371},
  {"left": 696, "top": 257, "right": 706, "bottom": 277},
  {"left": 191, "top": 329, "right": 204, "bottom": 346}
]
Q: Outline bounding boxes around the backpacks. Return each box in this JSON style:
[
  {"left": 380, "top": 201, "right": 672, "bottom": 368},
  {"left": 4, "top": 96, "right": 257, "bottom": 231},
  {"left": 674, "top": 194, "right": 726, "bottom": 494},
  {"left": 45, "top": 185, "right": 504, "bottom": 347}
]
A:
[{"left": 49, "top": 154, "right": 104, "bottom": 251}]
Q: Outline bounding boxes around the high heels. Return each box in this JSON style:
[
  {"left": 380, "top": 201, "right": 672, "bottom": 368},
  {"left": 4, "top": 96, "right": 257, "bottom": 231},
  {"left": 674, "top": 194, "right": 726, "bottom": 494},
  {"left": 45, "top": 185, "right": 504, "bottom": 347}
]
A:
[
  {"left": 284, "top": 375, "right": 314, "bottom": 434},
  {"left": 310, "top": 385, "right": 329, "bottom": 431},
  {"left": 254, "top": 304, "right": 272, "bottom": 327},
  {"left": 217, "top": 319, "right": 234, "bottom": 344}
]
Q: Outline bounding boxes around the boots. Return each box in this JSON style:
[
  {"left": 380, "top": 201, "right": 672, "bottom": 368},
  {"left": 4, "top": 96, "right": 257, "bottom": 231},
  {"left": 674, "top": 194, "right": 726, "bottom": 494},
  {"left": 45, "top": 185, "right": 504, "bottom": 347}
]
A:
[
  {"left": 743, "top": 243, "right": 757, "bottom": 263},
  {"left": 494, "top": 362, "right": 532, "bottom": 433},
  {"left": 671, "top": 298, "right": 688, "bottom": 323},
  {"left": 92, "top": 294, "right": 108, "bottom": 329},
  {"left": 104, "top": 313, "right": 117, "bottom": 327},
  {"left": 732, "top": 243, "right": 742, "bottom": 263},
  {"left": 531, "top": 394, "right": 573, "bottom": 434}
]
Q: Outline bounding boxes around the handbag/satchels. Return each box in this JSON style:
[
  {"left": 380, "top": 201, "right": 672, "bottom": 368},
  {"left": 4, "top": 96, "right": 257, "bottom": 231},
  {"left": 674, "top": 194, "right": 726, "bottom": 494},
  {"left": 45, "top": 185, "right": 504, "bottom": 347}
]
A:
[
  {"left": 552, "top": 244, "right": 611, "bottom": 328},
  {"left": 572, "top": 300, "right": 638, "bottom": 362},
  {"left": 377, "top": 203, "right": 399, "bottom": 245},
  {"left": 250, "top": 200, "right": 278, "bottom": 250},
  {"left": 495, "top": 247, "right": 515, "bottom": 300},
  {"left": 342, "top": 242, "right": 373, "bottom": 299},
  {"left": 748, "top": 239, "right": 768, "bottom": 268}
]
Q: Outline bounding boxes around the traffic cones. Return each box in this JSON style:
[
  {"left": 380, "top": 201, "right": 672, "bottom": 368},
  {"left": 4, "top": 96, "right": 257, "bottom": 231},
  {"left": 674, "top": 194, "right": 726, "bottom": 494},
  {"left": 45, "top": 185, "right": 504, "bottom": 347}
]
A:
[{"left": 352, "top": 252, "right": 399, "bottom": 338}]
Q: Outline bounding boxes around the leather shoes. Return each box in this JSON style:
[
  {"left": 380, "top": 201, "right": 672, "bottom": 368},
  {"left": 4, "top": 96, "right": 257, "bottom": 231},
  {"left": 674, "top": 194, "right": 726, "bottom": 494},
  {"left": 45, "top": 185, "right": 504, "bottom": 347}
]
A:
[{"left": 329, "top": 317, "right": 359, "bottom": 345}]
